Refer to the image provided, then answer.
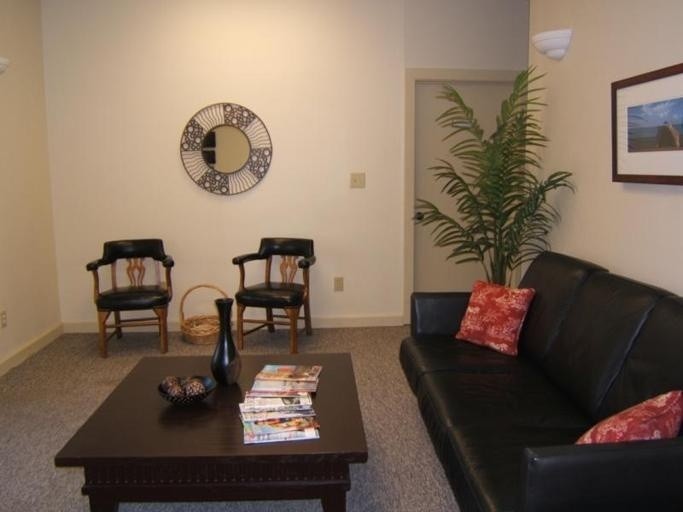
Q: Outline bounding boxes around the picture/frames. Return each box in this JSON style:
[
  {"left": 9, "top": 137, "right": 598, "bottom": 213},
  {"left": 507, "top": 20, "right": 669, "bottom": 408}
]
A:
[{"left": 611, "top": 64, "right": 683, "bottom": 185}]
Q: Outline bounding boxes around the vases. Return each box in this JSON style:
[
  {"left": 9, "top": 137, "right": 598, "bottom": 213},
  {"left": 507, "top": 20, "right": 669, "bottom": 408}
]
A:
[{"left": 210, "top": 297, "right": 241, "bottom": 386}]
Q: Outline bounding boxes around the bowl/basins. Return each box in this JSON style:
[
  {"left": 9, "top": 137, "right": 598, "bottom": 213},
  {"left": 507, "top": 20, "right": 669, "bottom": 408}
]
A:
[{"left": 156, "top": 374, "right": 219, "bottom": 409}]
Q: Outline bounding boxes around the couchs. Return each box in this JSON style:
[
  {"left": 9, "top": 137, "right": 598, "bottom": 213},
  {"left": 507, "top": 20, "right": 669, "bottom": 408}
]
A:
[{"left": 399, "top": 251, "right": 683, "bottom": 511}]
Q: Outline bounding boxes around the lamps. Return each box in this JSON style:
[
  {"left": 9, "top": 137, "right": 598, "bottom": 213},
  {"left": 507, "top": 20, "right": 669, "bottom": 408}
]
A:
[{"left": 531, "top": 28, "right": 573, "bottom": 61}]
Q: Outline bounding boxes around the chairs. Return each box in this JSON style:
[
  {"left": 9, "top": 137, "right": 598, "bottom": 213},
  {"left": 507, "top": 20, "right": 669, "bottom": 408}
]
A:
[
  {"left": 85, "top": 239, "right": 174, "bottom": 359},
  {"left": 231, "top": 237, "right": 317, "bottom": 356}
]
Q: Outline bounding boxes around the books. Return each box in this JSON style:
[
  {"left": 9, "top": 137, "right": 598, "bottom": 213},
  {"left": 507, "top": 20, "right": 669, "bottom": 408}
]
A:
[{"left": 238, "top": 361, "right": 323, "bottom": 447}]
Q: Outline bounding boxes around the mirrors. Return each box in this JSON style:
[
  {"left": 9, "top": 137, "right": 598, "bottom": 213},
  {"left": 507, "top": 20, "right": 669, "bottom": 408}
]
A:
[{"left": 179, "top": 103, "right": 272, "bottom": 196}]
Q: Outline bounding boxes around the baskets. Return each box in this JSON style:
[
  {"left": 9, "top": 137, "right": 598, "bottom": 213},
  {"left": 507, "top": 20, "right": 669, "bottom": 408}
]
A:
[{"left": 180, "top": 285, "right": 228, "bottom": 345}]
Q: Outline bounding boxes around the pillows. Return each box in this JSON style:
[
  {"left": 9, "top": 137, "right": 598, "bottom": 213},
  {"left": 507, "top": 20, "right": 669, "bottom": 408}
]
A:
[
  {"left": 451, "top": 280, "right": 538, "bottom": 357},
  {"left": 573, "top": 390, "right": 683, "bottom": 447}
]
